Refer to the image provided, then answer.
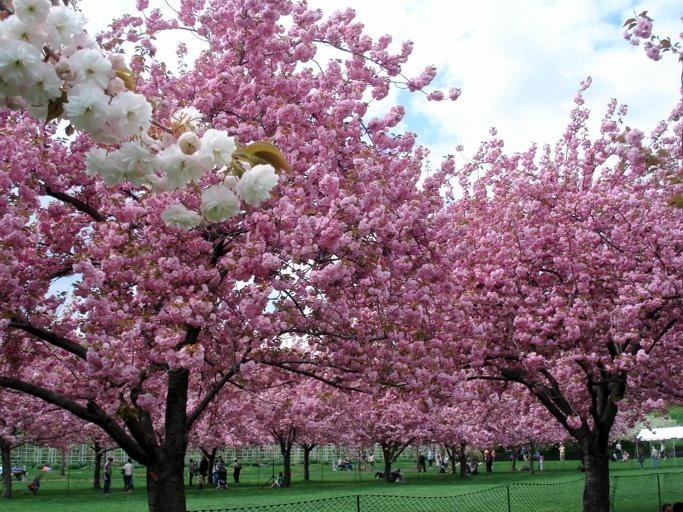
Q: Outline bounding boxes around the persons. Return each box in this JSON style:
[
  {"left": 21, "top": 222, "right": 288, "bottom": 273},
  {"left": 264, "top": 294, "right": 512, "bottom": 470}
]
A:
[
  {"left": 187, "top": 458, "right": 196, "bottom": 486},
  {"left": 216, "top": 465, "right": 229, "bottom": 489},
  {"left": 671, "top": 501, "right": 683, "bottom": 511},
  {"left": 465, "top": 462, "right": 478, "bottom": 473},
  {"left": 125, "top": 475, "right": 134, "bottom": 494},
  {"left": 661, "top": 502, "right": 672, "bottom": 511},
  {"left": 231, "top": 457, "right": 240, "bottom": 485},
  {"left": 538, "top": 452, "right": 544, "bottom": 471},
  {"left": 214, "top": 455, "right": 228, "bottom": 487},
  {"left": 557, "top": 444, "right": 565, "bottom": 461},
  {"left": 269, "top": 471, "right": 285, "bottom": 488},
  {"left": 490, "top": 447, "right": 495, "bottom": 462},
  {"left": 197, "top": 453, "right": 208, "bottom": 486},
  {"left": 439, "top": 459, "right": 446, "bottom": 473},
  {"left": 485, "top": 457, "right": 492, "bottom": 472},
  {"left": 609, "top": 439, "right": 668, "bottom": 469},
  {"left": 427, "top": 449, "right": 433, "bottom": 466},
  {"left": 121, "top": 457, "right": 133, "bottom": 493},
  {"left": 337, "top": 451, "right": 375, "bottom": 471},
  {"left": 378, "top": 468, "right": 400, "bottom": 482},
  {"left": 416, "top": 452, "right": 427, "bottom": 472},
  {"left": 101, "top": 456, "right": 112, "bottom": 494},
  {"left": 483, "top": 448, "right": 490, "bottom": 460}
]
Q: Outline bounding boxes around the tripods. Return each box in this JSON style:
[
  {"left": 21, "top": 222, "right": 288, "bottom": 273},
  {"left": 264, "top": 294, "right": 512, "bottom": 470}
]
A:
[{"left": 261, "top": 460, "right": 283, "bottom": 490}]
